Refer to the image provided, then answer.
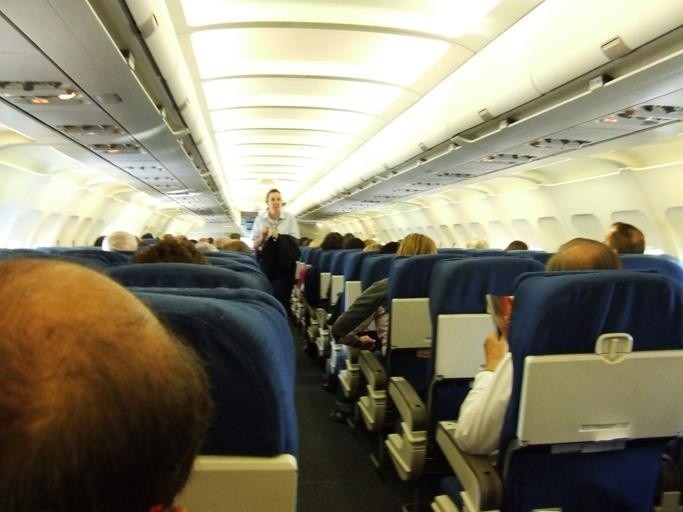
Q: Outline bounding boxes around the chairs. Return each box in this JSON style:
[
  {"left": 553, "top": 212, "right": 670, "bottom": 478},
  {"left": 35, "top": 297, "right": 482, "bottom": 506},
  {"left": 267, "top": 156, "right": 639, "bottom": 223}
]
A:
[
  {"left": 359, "top": 254, "right": 465, "bottom": 468},
  {"left": 431, "top": 269, "right": 682, "bottom": 512},
  {"left": 341, "top": 253, "right": 408, "bottom": 398},
  {"left": 428, "top": 245, "right": 559, "bottom": 263},
  {"left": 118, "top": 286, "right": 300, "bottom": 512},
  {"left": 291, "top": 240, "right": 362, "bottom": 396},
  {"left": 606, "top": 253, "right": 682, "bottom": 283},
  {"left": 389, "top": 256, "right": 547, "bottom": 482}
]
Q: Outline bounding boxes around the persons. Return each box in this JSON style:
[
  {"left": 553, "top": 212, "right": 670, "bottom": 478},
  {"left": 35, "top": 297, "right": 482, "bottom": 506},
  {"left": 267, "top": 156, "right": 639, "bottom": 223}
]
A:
[
  {"left": 250, "top": 189, "right": 301, "bottom": 316},
  {"left": 66, "top": 232, "right": 533, "bottom": 423},
  {"left": 1, "top": 255, "right": 218, "bottom": 512},
  {"left": 598, "top": 221, "right": 647, "bottom": 258},
  {"left": 401, "top": 237, "right": 624, "bottom": 512}
]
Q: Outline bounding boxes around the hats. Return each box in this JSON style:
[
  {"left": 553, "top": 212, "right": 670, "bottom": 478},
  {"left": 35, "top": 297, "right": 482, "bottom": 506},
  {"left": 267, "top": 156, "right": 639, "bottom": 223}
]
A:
[{"left": 102, "top": 231, "right": 138, "bottom": 253}]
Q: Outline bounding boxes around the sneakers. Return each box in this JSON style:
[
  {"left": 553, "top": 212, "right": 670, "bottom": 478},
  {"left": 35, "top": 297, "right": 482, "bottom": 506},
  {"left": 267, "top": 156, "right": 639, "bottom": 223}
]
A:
[{"left": 328, "top": 406, "right": 350, "bottom": 423}]
